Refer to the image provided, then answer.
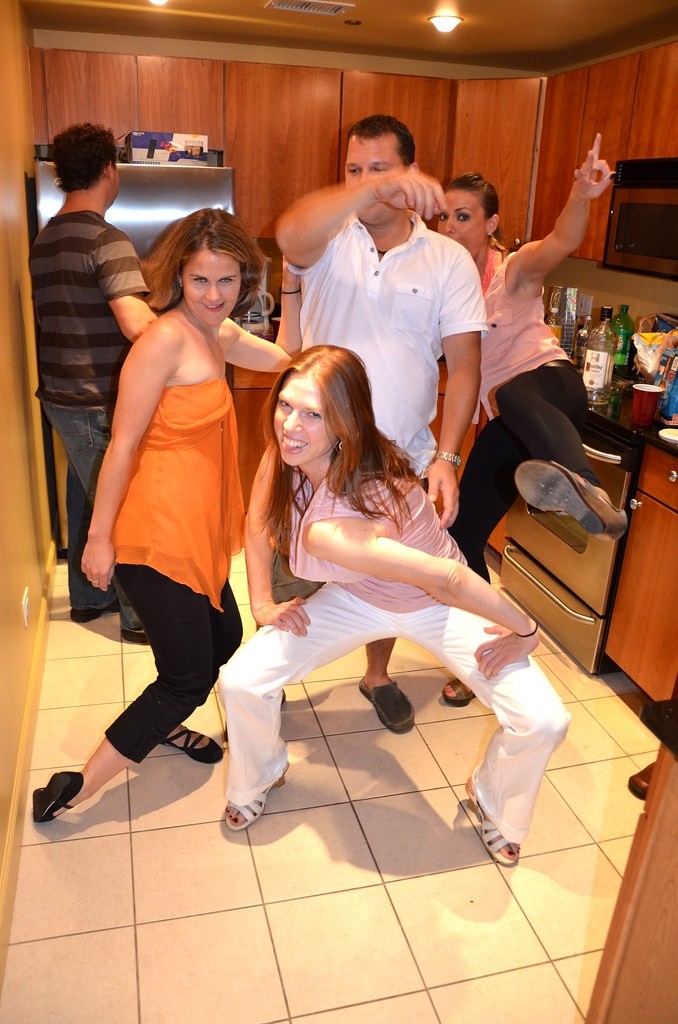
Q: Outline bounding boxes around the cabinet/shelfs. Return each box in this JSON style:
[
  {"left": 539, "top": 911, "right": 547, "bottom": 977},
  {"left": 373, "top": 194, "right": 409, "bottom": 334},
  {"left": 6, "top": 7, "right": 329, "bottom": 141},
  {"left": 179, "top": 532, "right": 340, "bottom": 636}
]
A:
[{"left": 34, "top": 31, "right": 678, "bottom": 699}]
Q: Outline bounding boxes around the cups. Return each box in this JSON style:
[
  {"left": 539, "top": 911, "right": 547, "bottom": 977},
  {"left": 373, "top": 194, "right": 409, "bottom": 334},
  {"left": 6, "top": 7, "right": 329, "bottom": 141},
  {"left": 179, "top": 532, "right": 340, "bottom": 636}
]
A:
[{"left": 631, "top": 384, "right": 664, "bottom": 427}]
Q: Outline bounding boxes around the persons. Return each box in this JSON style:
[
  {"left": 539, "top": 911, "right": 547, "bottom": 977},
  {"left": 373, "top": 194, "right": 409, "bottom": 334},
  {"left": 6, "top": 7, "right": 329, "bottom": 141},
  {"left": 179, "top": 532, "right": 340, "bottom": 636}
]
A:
[
  {"left": 219, "top": 347, "right": 571, "bottom": 867},
  {"left": 27, "top": 124, "right": 160, "bottom": 645},
  {"left": 438, "top": 133, "right": 627, "bottom": 707},
  {"left": 276, "top": 113, "right": 489, "bottom": 734},
  {"left": 33, "top": 207, "right": 302, "bottom": 821}
]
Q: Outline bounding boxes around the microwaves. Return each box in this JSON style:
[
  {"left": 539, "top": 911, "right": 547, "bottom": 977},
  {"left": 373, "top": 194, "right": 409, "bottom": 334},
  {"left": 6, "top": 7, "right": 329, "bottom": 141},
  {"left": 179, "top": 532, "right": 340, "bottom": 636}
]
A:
[{"left": 603, "top": 158, "right": 678, "bottom": 279}]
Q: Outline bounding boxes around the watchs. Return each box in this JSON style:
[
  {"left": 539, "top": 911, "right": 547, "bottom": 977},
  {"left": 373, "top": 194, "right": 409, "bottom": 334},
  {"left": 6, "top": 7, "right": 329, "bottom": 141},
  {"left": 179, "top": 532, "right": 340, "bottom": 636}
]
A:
[{"left": 437, "top": 452, "right": 461, "bottom": 469}]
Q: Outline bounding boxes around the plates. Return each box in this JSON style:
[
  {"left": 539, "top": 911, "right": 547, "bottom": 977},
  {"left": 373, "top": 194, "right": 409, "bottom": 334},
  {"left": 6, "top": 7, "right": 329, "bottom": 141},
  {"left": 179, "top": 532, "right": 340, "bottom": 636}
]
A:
[{"left": 657, "top": 428, "right": 678, "bottom": 443}]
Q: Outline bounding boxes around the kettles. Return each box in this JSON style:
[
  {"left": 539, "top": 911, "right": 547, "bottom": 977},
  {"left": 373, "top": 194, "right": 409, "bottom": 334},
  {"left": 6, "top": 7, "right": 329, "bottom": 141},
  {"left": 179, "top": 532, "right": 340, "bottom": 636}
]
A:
[{"left": 234, "top": 286, "right": 275, "bottom": 333}]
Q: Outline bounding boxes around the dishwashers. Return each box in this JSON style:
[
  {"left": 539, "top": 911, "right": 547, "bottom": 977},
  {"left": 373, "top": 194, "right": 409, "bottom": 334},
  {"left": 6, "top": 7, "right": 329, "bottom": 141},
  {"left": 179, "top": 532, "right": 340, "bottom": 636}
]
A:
[{"left": 503, "top": 407, "right": 635, "bottom": 675}]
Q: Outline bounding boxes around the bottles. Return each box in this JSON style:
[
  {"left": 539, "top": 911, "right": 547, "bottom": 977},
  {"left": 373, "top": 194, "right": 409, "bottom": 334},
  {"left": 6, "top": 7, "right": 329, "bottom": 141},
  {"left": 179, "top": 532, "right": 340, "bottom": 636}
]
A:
[
  {"left": 545, "top": 307, "right": 562, "bottom": 347},
  {"left": 573, "top": 304, "right": 634, "bottom": 404}
]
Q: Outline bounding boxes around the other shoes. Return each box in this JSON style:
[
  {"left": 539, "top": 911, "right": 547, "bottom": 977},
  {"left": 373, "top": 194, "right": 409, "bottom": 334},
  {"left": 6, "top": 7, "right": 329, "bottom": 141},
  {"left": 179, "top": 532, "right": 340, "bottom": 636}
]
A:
[
  {"left": 32, "top": 771, "right": 84, "bottom": 823},
  {"left": 160, "top": 726, "right": 224, "bottom": 764},
  {"left": 629, "top": 762, "right": 656, "bottom": 798},
  {"left": 69, "top": 597, "right": 120, "bottom": 623},
  {"left": 358, "top": 675, "right": 415, "bottom": 734},
  {"left": 121, "top": 627, "right": 148, "bottom": 643}
]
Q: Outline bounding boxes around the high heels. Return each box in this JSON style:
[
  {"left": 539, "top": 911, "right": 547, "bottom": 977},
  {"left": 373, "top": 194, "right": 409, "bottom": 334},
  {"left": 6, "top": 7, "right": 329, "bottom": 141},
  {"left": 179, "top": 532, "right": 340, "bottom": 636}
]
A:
[
  {"left": 225, "top": 762, "right": 290, "bottom": 832},
  {"left": 465, "top": 782, "right": 520, "bottom": 867},
  {"left": 443, "top": 678, "right": 478, "bottom": 707},
  {"left": 513, "top": 459, "right": 627, "bottom": 543}
]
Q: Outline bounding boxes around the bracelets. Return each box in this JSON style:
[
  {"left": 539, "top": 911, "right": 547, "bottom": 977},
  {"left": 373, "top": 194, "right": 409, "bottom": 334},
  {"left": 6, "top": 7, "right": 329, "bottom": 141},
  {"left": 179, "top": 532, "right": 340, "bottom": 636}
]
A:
[
  {"left": 516, "top": 621, "right": 540, "bottom": 638},
  {"left": 281, "top": 289, "right": 301, "bottom": 295}
]
